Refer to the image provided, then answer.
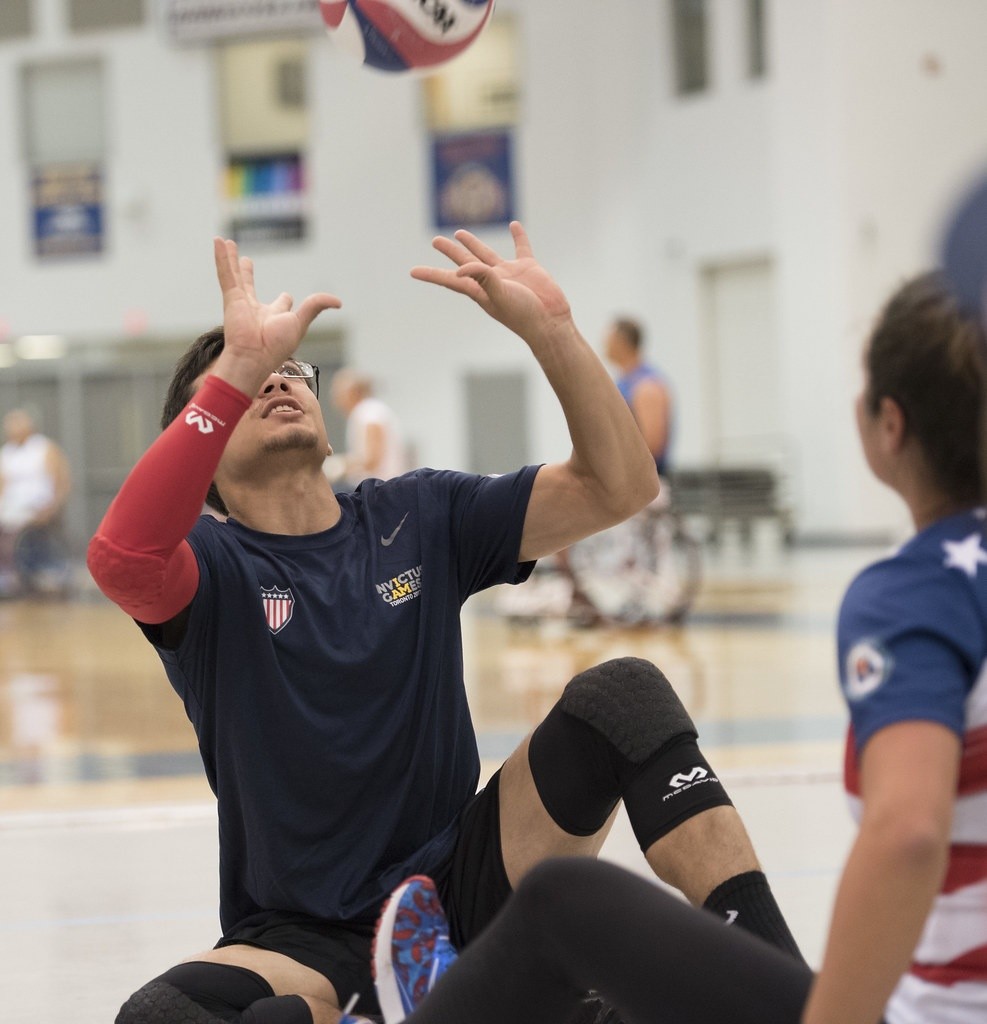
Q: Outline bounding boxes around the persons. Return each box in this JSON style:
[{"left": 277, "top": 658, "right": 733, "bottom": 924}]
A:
[
  {"left": 0, "top": 406, "right": 68, "bottom": 591},
  {"left": 557, "top": 314, "right": 670, "bottom": 569},
  {"left": 87, "top": 219, "right": 810, "bottom": 1024},
  {"left": 373, "top": 269, "right": 986, "bottom": 1024},
  {"left": 321, "top": 366, "right": 405, "bottom": 490}
]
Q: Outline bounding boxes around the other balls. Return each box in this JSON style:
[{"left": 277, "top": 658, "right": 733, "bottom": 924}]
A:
[{"left": 316, "top": 0, "right": 496, "bottom": 74}]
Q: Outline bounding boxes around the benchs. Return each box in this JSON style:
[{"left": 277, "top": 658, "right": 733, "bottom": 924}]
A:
[{"left": 662, "top": 468, "right": 794, "bottom": 547}]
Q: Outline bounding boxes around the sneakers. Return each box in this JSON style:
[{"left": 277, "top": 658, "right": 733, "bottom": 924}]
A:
[{"left": 369, "top": 874, "right": 459, "bottom": 1024}]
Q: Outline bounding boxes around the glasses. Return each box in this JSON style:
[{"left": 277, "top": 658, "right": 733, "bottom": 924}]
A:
[{"left": 274, "top": 356, "right": 320, "bottom": 401}]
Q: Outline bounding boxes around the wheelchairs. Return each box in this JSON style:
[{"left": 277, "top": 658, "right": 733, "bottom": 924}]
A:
[
  {"left": 495, "top": 480, "right": 702, "bottom": 632},
  {"left": 4, "top": 514, "right": 77, "bottom": 597}
]
